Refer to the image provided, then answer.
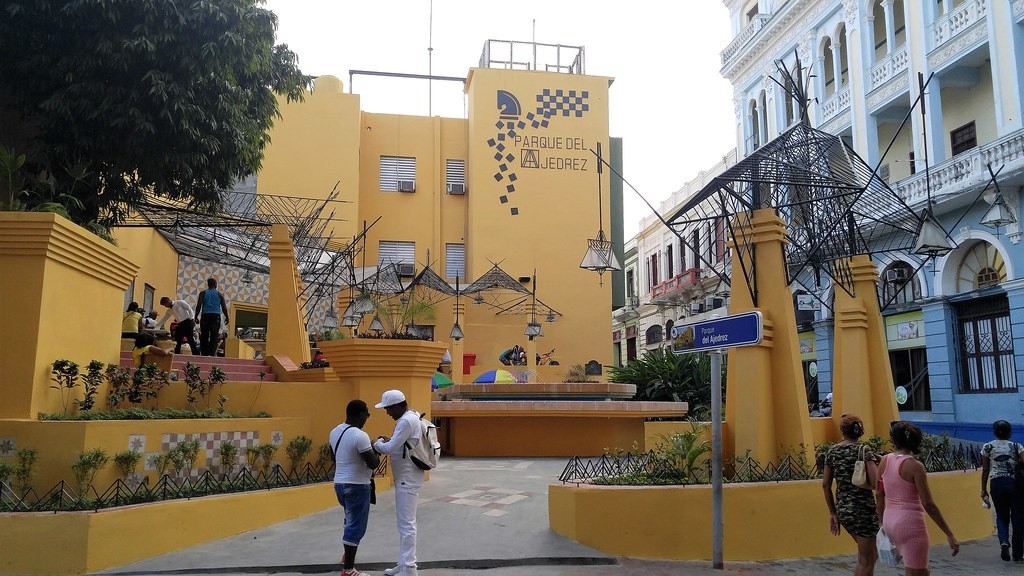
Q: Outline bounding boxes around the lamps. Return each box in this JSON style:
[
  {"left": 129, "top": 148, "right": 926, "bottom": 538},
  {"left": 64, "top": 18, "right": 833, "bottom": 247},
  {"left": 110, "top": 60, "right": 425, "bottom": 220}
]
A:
[
  {"left": 314, "top": 285, "right": 326, "bottom": 299},
  {"left": 400, "top": 292, "right": 409, "bottom": 305},
  {"left": 368, "top": 266, "right": 385, "bottom": 331},
  {"left": 340, "top": 220, "right": 376, "bottom": 338},
  {"left": 240, "top": 268, "right": 253, "bottom": 286},
  {"left": 449, "top": 270, "right": 464, "bottom": 347},
  {"left": 907, "top": 72, "right": 959, "bottom": 275},
  {"left": 545, "top": 309, "right": 556, "bottom": 325},
  {"left": 523, "top": 269, "right": 544, "bottom": 342},
  {"left": 472, "top": 290, "right": 486, "bottom": 307},
  {"left": 712, "top": 217, "right": 731, "bottom": 309},
  {"left": 979, "top": 164, "right": 1017, "bottom": 240},
  {"left": 579, "top": 142, "right": 622, "bottom": 287}
]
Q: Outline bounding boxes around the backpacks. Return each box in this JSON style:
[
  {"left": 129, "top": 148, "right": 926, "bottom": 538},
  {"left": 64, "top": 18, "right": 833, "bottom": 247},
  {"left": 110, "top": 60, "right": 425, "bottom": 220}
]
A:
[{"left": 397, "top": 411, "right": 441, "bottom": 471}]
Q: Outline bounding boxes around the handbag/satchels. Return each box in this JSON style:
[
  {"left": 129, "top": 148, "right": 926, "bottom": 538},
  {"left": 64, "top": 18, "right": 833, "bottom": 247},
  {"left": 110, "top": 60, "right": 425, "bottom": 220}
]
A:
[
  {"left": 1014, "top": 443, "right": 1024, "bottom": 494},
  {"left": 852, "top": 445, "right": 876, "bottom": 490},
  {"left": 876, "top": 526, "right": 903, "bottom": 567}
]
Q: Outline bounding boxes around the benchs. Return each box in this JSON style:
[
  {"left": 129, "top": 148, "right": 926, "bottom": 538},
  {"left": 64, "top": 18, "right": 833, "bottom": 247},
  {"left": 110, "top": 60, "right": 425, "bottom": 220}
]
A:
[
  {"left": 121, "top": 338, "right": 177, "bottom": 355},
  {"left": 439, "top": 364, "right": 451, "bottom": 374}
]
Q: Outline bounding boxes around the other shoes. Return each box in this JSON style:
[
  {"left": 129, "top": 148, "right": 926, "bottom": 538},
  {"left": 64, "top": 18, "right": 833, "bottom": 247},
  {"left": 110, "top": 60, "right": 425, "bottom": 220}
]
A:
[
  {"left": 1001, "top": 545, "right": 1010, "bottom": 561},
  {"left": 393, "top": 567, "right": 418, "bottom": 576},
  {"left": 342, "top": 553, "right": 345, "bottom": 564},
  {"left": 340, "top": 568, "right": 370, "bottom": 576},
  {"left": 1013, "top": 557, "right": 1024, "bottom": 563},
  {"left": 385, "top": 563, "right": 400, "bottom": 575}
]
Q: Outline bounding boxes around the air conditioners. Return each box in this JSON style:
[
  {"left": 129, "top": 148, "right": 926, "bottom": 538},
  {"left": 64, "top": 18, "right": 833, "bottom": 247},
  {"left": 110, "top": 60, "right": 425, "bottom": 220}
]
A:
[
  {"left": 401, "top": 180, "right": 415, "bottom": 192},
  {"left": 449, "top": 183, "right": 466, "bottom": 195},
  {"left": 399, "top": 265, "right": 414, "bottom": 275},
  {"left": 887, "top": 269, "right": 907, "bottom": 282}
]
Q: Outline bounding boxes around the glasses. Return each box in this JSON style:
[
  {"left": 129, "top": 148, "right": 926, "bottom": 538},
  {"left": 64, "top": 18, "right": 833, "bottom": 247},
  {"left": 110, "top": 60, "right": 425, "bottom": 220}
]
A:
[{"left": 364, "top": 413, "right": 370, "bottom": 417}]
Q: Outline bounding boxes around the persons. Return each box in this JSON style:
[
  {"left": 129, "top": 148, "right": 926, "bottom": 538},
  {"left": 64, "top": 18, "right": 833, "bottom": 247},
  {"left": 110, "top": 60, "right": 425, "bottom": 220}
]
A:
[
  {"left": 817, "top": 387, "right": 833, "bottom": 417},
  {"left": 122, "top": 278, "right": 229, "bottom": 381},
  {"left": 328, "top": 399, "right": 381, "bottom": 576},
  {"left": 875, "top": 421, "right": 960, "bottom": 576},
  {"left": 371, "top": 389, "right": 425, "bottom": 576},
  {"left": 980, "top": 420, "right": 1024, "bottom": 562},
  {"left": 821, "top": 413, "right": 879, "bottom": 576}
]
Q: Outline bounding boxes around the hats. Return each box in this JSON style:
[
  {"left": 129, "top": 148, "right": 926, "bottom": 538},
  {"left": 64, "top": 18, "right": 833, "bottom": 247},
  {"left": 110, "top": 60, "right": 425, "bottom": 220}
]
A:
[{"left": 375, "top": 390, "right": 405, "bottom": 408}]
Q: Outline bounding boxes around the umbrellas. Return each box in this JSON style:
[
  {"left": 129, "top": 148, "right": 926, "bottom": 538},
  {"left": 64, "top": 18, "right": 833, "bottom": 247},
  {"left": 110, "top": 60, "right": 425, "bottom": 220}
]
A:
[
  {"left": 472, "top": 369, "right": 519, "bottom": 383},
  {"left": 432, "top": 372, "right": 454, "bottom": 389}
]
